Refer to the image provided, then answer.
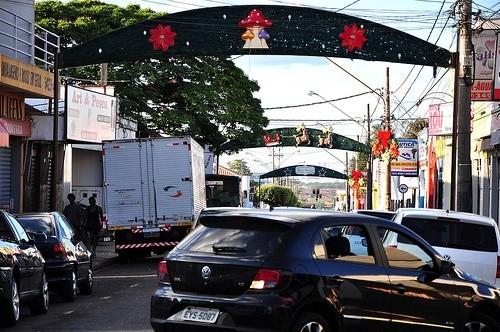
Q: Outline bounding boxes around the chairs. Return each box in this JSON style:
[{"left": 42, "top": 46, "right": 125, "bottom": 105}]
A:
[{"left": 325, "top": 235, "right": 350, "bottom": 260}]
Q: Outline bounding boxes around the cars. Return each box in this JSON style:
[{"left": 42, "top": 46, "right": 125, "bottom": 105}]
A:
[
  {"left": 12, "top": 211, "right": 95, "bottom": 302},
  {"left": 361, "top": 207, "right": 500, "bottom": 289},
  {"left": 341, "top": 208, "right": 396, "bottom": 258},
  {"left": 0, "top": 208, "right": 50, "bottom": 327}
]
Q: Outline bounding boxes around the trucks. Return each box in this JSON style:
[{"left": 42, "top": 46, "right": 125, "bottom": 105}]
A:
[{"left": 101, "top": 135, "right": 211, "bottom": 262}]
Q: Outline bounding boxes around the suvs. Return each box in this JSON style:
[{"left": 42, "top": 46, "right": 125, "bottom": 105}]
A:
[{"left": 149, "top": 206, "right": 500, "bottom": 332}]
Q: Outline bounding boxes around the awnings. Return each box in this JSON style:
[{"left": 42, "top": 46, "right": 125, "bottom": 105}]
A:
[
  {"left": 2, "top": 117, "right": 31, "bottom": 137},
  {"left": 474, "top": 129, "right": 500, "bottom": 153},
  {"left": 0, "top": 121, "right": 9, "bottom": 147}
]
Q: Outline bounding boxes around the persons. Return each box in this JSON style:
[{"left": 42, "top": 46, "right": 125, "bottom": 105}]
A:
[
  {"left": 84, "top": 197, "right": 103, "bottom": 256},
  {"left": 64, "top": 193, "right": 87, "bottom": 237}
]
